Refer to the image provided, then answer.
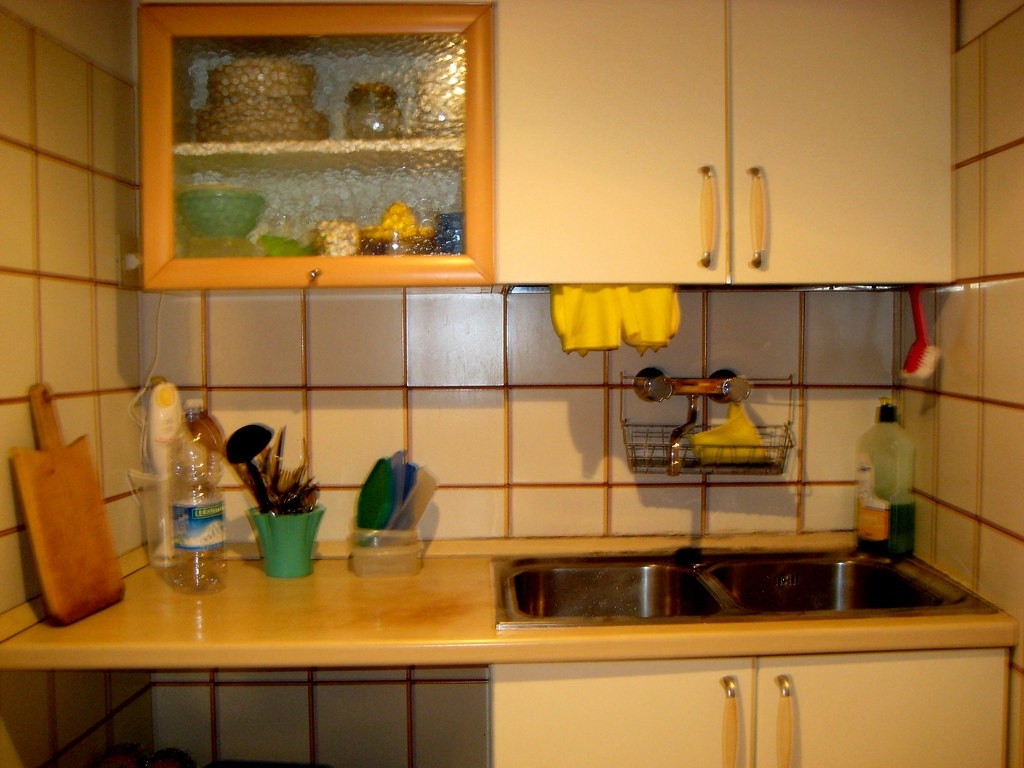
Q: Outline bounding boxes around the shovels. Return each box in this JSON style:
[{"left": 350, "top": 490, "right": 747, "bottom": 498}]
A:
[{"left": 187, "top": 412, "right": 258, "bottom": 507}]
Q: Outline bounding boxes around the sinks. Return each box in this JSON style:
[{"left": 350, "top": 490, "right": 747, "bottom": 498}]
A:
[
  {"left": 493, "top": 565, "right": 722, "bottom": 630},
  {"left": 709, "top": 561, "right": 999, "bottom": 623}
]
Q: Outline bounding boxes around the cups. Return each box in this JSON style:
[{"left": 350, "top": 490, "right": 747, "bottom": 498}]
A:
[
  {"left": 435, "top": 211, "right": 463, "bottom": 252},
  {"left": 249, "top": 506, "right": 326, "bottom": 578}
]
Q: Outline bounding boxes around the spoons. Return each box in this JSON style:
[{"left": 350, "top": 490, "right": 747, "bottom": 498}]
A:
[{"left": 184, "top": 406, "right": 264, "bottom": 509}]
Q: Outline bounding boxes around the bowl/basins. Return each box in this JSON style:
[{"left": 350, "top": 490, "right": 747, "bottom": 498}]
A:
[
  {"left": 179, "top": 184, "right": 265, "bottom": 236},
  {"left": 359, "top": 226, "right": 435, "bottom": 254},
  {"left": 349, "top": 518, "right": 422, "bottom": 576}
]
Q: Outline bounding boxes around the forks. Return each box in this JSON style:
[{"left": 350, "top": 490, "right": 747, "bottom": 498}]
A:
[{"left": 241, "top": 426, "right": 320, "bottom": 514}]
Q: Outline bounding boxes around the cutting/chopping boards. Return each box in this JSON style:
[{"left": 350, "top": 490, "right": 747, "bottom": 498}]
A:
[{"left": 10, "top": 384, "right": 125, "bottom": 626}]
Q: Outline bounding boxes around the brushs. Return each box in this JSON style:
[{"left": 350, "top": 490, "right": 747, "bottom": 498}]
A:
[{"left": 900, "top": 286, "right": 940, "bottom": 378}]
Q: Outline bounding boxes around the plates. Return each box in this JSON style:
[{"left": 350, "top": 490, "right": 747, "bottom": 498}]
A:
[{"left": 193, "top": 59, "right": 328, "bottom": 138}]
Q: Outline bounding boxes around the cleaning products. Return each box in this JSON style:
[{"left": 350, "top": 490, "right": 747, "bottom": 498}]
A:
[{"left": 853, "top": 401, "right": 916, "bottom": 557}]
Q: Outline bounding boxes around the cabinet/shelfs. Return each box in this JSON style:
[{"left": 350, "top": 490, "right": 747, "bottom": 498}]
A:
[
  {"left": 487, "top": 649, "right": 1010, "bottom": 768},
  {"left": 132, "top": 0, "right": 957, "bottom": 293}
]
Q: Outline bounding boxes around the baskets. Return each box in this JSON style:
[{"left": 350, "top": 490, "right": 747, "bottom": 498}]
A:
[{"left": 623, "top": 424, "right": 793, "bottom": 475}]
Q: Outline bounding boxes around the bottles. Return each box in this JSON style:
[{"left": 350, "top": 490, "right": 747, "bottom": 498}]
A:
[
  {"left": 167, "top": 400, "right": 227, "bottom": 594},
  {"left": 345, "top": 81, "right": 396, "bottom": 136}
]
